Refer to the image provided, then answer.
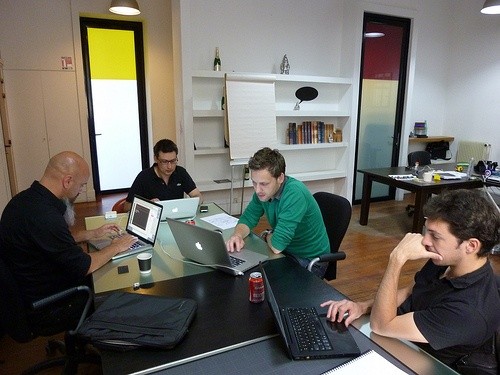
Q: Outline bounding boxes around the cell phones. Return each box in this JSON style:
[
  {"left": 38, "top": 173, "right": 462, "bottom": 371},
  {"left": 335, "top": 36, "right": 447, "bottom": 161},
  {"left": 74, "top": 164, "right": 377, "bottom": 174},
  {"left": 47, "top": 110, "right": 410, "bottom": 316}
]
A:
[{"left": 201, "top": 206, "right": 208, "bottom": 212}]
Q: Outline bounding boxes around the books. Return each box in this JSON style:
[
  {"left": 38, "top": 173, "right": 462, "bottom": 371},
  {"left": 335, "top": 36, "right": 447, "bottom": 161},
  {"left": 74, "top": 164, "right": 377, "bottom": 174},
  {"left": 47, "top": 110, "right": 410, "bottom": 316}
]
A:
[
  {"left": 286, "top": 121, "right": 334, "bottom": 144},
  {"left": 414, "top": 120, "right": 427, "bottom": 135}
]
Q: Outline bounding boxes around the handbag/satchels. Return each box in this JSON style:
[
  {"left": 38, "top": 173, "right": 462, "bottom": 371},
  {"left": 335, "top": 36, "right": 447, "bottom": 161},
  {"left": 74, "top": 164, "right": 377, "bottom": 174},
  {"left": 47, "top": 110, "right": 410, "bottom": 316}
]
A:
[{"left": 76, "top": 291, "right": 198, "bottom": 350}]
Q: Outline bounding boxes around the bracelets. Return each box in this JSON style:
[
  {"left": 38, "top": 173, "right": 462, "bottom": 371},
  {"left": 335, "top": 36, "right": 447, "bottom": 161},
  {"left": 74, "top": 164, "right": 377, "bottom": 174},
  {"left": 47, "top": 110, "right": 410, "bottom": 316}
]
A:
[{"left": 265, "top": 231, "right": 273, "bottom": 241}]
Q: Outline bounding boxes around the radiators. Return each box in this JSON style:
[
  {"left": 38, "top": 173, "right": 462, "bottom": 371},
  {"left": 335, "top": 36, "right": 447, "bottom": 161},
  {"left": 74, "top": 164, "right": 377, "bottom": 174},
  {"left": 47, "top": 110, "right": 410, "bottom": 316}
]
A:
[{"left": 456, "top": 141, "right": 491, "bottom": 164}]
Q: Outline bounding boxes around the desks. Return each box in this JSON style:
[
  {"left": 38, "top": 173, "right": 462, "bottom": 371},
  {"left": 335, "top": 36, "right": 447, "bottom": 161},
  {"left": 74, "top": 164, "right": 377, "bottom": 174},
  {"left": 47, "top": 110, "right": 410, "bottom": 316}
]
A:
[
  {"left": 356, "top": 162, "right": 491, "bottom": 236},
  {"left": 84, "top": 202, "right": 459, "bottom": 375}
]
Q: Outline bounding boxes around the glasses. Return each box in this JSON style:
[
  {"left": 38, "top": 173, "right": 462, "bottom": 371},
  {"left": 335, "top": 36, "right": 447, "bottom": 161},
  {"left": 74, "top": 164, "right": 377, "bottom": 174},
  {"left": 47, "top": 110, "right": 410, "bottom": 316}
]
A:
[{"left": 157, "top": 156, "right": 177, "bottom": 165}]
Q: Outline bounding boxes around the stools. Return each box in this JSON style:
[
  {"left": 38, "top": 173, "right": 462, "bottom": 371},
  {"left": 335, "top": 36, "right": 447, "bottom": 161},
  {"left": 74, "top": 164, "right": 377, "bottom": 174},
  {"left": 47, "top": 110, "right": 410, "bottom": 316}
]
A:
[{"left": 480, "top": 0, "right": 500, "bottom": 15}]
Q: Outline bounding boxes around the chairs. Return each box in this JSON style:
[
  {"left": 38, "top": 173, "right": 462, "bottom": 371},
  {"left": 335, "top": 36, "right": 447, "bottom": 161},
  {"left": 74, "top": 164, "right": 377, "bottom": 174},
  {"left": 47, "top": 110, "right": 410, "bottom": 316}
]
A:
[
  {"left": 0, "top": 259, "right": 101, "bottom": 375},
  {"left": 306, "top": 192, "right": 353, "bottom": 282},
  {"left": 405, "top": 151, "right": 433, "bottom": 218}
]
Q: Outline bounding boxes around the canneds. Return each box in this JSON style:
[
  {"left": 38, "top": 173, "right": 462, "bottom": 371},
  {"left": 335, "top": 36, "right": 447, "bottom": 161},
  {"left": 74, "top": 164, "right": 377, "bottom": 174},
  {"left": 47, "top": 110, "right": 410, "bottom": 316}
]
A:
[
  {"left": 185, "top": 219, "right": 195, "bottom": 225},
  {"left": 248, "top": 272, "right": 265, "bottom": 303}
]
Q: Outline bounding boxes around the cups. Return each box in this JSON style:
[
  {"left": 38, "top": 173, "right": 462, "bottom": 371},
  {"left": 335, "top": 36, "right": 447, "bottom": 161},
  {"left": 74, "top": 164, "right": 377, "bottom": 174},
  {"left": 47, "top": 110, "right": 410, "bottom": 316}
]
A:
[
  {"left": 424, "top": 172, "right": 433, "bottom": 182},
  {"left": 139, "top": 272, "right": 155, "bottom": 289},
  {"left": 136, "top": 253, "right": 152, "bottom": 273}
]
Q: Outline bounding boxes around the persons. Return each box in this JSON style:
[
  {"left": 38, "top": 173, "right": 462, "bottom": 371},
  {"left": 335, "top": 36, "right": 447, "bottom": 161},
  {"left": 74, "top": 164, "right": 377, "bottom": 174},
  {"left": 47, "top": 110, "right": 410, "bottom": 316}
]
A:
[
  {"left": 225, "top": 147, "right": 330, "bottom": 278},
  {"left": 320, "top": 189, "right": 500, "bottom": 375},
  {"left": 0, "top": 151, "right": 139, "bottom": 338},
  {"left": 123, "top": 139, "right": 203, "bottom": 212}
]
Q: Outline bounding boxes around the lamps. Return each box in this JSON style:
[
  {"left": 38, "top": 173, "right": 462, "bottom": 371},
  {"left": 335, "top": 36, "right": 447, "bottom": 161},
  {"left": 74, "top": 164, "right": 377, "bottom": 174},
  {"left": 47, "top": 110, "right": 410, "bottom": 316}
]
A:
[{"left": 109, "top": 0, "right": 142, "bottom": 16}]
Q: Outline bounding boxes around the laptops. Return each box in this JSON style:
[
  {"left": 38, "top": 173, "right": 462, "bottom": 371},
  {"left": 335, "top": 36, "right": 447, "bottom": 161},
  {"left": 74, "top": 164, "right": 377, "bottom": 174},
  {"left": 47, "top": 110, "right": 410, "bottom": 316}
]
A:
[
  {"left": 158, "top": 197, "right": 201, "bottom": 222},
  {"left": 259, "top": 261, "right": 362, "bottom": 361},
  {"left": 166, "top": 217, "right": 274, "bottom": 276},
  {"left": 87, "top": 195, "right": 163, "bottom": 261}
]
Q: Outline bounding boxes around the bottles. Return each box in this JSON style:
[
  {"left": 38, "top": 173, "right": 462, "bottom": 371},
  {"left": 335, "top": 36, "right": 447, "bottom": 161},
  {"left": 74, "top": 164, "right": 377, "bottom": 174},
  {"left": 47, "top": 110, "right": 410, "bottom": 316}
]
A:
[
  {"left": 244, "top": 165, "right": 249, "bottom": 180},
  {"left": 213, "top": 47, "right": 222, "bottom": 71}
]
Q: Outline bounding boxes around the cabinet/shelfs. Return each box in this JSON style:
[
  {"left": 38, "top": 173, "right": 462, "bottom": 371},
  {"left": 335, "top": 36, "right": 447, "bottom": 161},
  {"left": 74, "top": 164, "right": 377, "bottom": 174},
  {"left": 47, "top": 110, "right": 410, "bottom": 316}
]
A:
[{"left": 192, "top": 68, "right": 353, "bottom": 191}]
